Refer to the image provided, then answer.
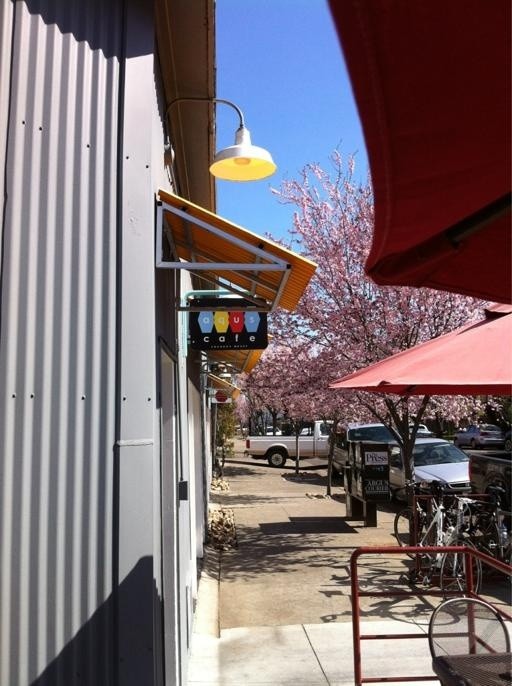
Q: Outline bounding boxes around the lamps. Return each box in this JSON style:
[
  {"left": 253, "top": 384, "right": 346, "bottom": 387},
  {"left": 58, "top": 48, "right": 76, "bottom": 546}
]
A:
[{"left": 162, "top": 97, "right": 276, "bottom": 181}]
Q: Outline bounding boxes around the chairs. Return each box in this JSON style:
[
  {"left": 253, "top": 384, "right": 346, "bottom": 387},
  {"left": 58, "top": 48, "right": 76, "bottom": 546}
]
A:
[{"left": 429, "top": 598, "right": 510, "bottom": 658}]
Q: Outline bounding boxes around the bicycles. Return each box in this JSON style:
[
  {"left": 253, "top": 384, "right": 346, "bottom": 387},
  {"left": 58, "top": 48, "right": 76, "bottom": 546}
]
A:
[{"left": 393, "top": 480, "right": 508, "bottom": 600}]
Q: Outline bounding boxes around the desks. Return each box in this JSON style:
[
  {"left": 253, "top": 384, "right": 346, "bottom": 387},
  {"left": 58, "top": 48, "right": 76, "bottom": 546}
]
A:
[{"left": 431, "top": 652, "right": 512, "bottom": 686}]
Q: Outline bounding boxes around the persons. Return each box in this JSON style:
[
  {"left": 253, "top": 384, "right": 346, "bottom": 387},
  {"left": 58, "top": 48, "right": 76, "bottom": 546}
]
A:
[
  {"left": 242, "top": 423, "right": 263, "bottom": 439},
  {"left": 274, "top": 415, "right": 314, "bottom": 436}
]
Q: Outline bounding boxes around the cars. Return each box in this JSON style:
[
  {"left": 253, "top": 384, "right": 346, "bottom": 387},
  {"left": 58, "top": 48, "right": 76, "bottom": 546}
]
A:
[
  {"left": 453, "top": 424, "right": 504, "bottom": 449},
  {"left": 258, "top": 425, "right": 282, "bottom": 436},
  {"left": 332, "top": 424, "right": 401, "bottom": 476},
  {"left": 387, "top": 437, "right": 471, "bottom": 502},
  {"left": 409, "top": 422, "right": 433, "bottom": 437}
]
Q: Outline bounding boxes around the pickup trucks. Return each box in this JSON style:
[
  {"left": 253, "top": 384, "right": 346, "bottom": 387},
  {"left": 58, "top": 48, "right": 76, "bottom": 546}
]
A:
[{"left": 245, "top": 420, "right": 343, "bottom": 467}]
